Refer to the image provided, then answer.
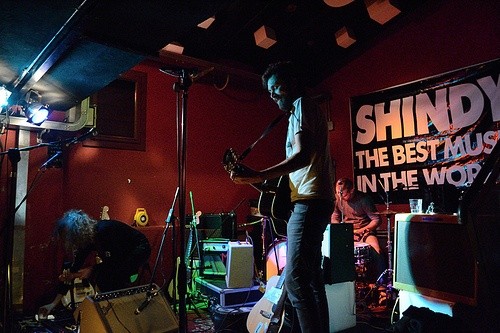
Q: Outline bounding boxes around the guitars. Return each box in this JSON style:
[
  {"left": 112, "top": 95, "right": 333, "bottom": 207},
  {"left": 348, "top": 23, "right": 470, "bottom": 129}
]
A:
[
  {"left": 57, "top": 268, "right": 82, "bottom": 282},
  {"left": 167, "top": 210, "right": 202, "bottom": 301},
  {"left": 96, "top": 205, "right": 114, "bottom": 265},
  {"left": 246, "top": 266, "right": 286, "bottom": 333},
  {"left": 220, "top": 145, "right": 296, "bottom": 238}
]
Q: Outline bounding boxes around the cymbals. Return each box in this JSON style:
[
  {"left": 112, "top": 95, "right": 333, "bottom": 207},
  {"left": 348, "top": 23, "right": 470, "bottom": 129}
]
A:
[{"left": 369, "top": 209, "right": 405, "bottom": 215}]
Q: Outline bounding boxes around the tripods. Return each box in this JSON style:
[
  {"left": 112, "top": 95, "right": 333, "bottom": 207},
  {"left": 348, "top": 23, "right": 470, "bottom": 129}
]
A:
[{"left": 355, "top": 177, "right": 398, "bottom": 309}]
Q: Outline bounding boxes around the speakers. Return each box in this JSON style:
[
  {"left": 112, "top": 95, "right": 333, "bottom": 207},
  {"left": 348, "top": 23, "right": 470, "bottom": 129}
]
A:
[
  {"left": 184, "top": 211, "right": 237, "bottom": 259},
  {"left": 392, "top": 212, "right": 477, "bottom": 306},
  {"left": 321, "top": 222, "right": 355, "bottom": 284},
  {"left": 73, "top": 283, "right": 179, "bottom": 333}
]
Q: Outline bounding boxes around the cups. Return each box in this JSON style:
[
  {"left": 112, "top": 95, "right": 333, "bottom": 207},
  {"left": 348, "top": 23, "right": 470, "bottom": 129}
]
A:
[{"left": 409, "top": 198, "right": 423, "bottom": 213}]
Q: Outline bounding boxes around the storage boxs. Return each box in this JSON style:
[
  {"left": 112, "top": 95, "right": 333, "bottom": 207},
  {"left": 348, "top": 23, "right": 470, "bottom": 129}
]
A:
[{"left": 195, "top": 278, "right": 266, "bottom": 308}]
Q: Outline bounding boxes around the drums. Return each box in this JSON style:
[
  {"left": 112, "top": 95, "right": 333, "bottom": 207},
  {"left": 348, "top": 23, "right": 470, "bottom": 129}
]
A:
[
  {"left": 261, "top": 237, "right": 288, "bottom": 281},
  {"left": 353, "top": 242, "right": 377, "bottom": 281}
]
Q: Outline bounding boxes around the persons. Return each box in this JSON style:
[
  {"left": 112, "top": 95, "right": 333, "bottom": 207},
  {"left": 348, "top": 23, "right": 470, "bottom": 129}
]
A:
[
  {"left": 230, "top": 61, "right": 336, "bottom": 333},
  {"left": 331, "top": 178, "right": 390, "bottom": 304},
  {"left": 38, "top": 210, "right": 151, "bottom": 317}
]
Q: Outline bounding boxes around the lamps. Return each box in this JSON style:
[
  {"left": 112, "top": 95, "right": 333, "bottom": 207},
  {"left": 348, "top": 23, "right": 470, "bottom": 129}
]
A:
[{"left": 25, "top": 100, "right": 54, "bottom": 127}]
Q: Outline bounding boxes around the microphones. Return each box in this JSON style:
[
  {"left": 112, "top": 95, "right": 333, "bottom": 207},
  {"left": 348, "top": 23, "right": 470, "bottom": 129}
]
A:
[
  {"left": 135, "top": 292, "right": 156, "bottom": 314},
  {"left": 37, "top": 151, "right": 64, "bottom": 172},
  {"left": 65, "top": 127, "right": 98, "bottom": 147}
]
